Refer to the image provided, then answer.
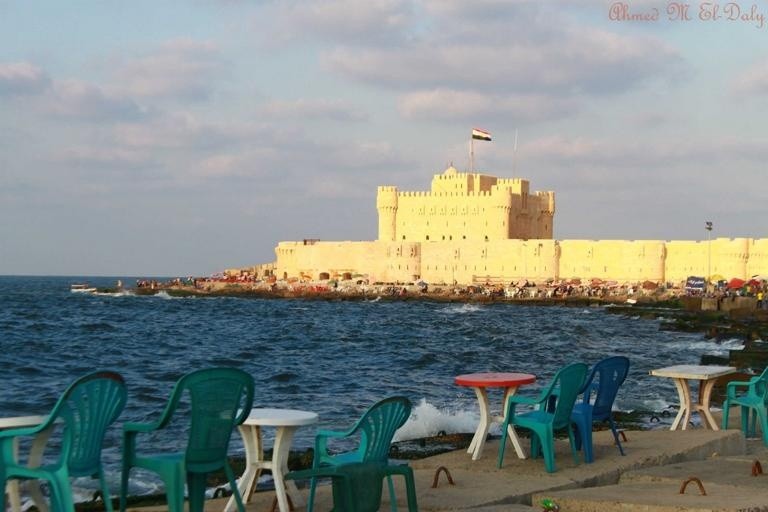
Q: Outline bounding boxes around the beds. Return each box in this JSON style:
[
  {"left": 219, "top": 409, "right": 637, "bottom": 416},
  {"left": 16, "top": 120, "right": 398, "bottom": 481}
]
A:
[{"left": 472, "top": 128, "right": 491, "bottom": 141}]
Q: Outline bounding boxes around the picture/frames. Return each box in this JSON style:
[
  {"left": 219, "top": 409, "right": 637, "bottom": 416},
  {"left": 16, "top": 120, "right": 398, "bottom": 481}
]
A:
[
  {"left": 648, "top": 364, "right": 736, "bottom": 432},
  {"left": 219, "top": 409, "right": 319, "bottom": 512},
  {"left": 0, "top": 415, "right": 64, "bottom": 512},
  {"left": 455, "top": 373, "right": 536, "bottom": 460}
]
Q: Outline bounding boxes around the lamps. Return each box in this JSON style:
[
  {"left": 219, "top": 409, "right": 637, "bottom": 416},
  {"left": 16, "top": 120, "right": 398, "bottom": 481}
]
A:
[
  {"left": 751, "top": 382, "right": 764, "bottom": 438},
  {"left": 307, "top": 395, "right": 411, "bottom": 512},
  {"left": 0, "top": 370, "right": 128, "bottom": 512},
  {"left": 120, "top": 368, "right": 254, "bottom": 512},
  {"left": 547, "top": 356, "right": 629, "bottom": 462},
  {"left": 497, "top": 363, "right": 589, "bottom": 473},
  {"left": 283, "top": 464, "right": 419, "bottom": 512},
  {"left": 721, "top": 366, "right": 768, "bottom": 449}
]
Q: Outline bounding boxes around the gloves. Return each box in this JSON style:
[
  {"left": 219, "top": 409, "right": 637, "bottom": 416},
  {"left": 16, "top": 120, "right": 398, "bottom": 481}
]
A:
[{"left": 70, "top": 283, "right": 97, "bottom": 292}]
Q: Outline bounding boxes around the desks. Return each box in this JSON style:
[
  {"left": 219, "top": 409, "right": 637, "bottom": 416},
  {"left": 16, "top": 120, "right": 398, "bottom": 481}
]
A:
[{"left": 705, "top": 221, "right": 712, "bottom": 284}]
